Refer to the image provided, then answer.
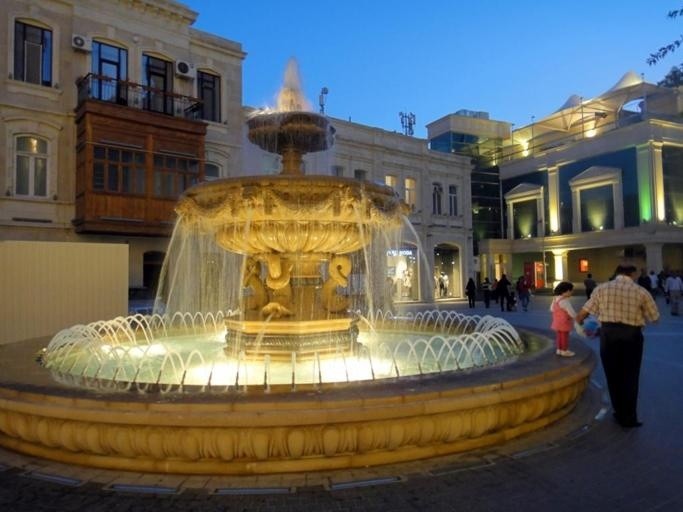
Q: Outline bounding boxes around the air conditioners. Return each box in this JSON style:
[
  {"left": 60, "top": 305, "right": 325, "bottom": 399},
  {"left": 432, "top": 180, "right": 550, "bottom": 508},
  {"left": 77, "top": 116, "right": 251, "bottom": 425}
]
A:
[
  {"left": 70, "top": 33, "right": 92, "bottom": 53},
  {"left": 174, "top": 60, "right": 195, "bottom": 82}
]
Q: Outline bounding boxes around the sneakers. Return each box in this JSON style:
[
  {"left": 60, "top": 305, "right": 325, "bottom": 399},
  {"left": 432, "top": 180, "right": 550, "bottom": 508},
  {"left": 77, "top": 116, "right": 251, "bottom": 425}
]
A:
[{"left": 556, "top": 349, "right": 575, "bottom": 357}]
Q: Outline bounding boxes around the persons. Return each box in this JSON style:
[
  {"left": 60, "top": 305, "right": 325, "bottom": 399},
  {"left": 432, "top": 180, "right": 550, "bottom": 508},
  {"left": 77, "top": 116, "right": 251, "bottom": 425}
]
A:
[
  {"left": 576, "top": 264, "right": 660, "bottom": 427},
  {"left": 550, "top": 282, "right": 578, "bottom": 357},
  {"left": 434, "top": 272, "right": 449, "bottom": 297},
  {"left": 466, "top": 275, "right": 532, "bottom": 311},
  {"left": 609, "top": 266, "right": 683, "bottom": 316},
  {"left": 584, "top": 274, "right": 597, "bottom": 299}
]
